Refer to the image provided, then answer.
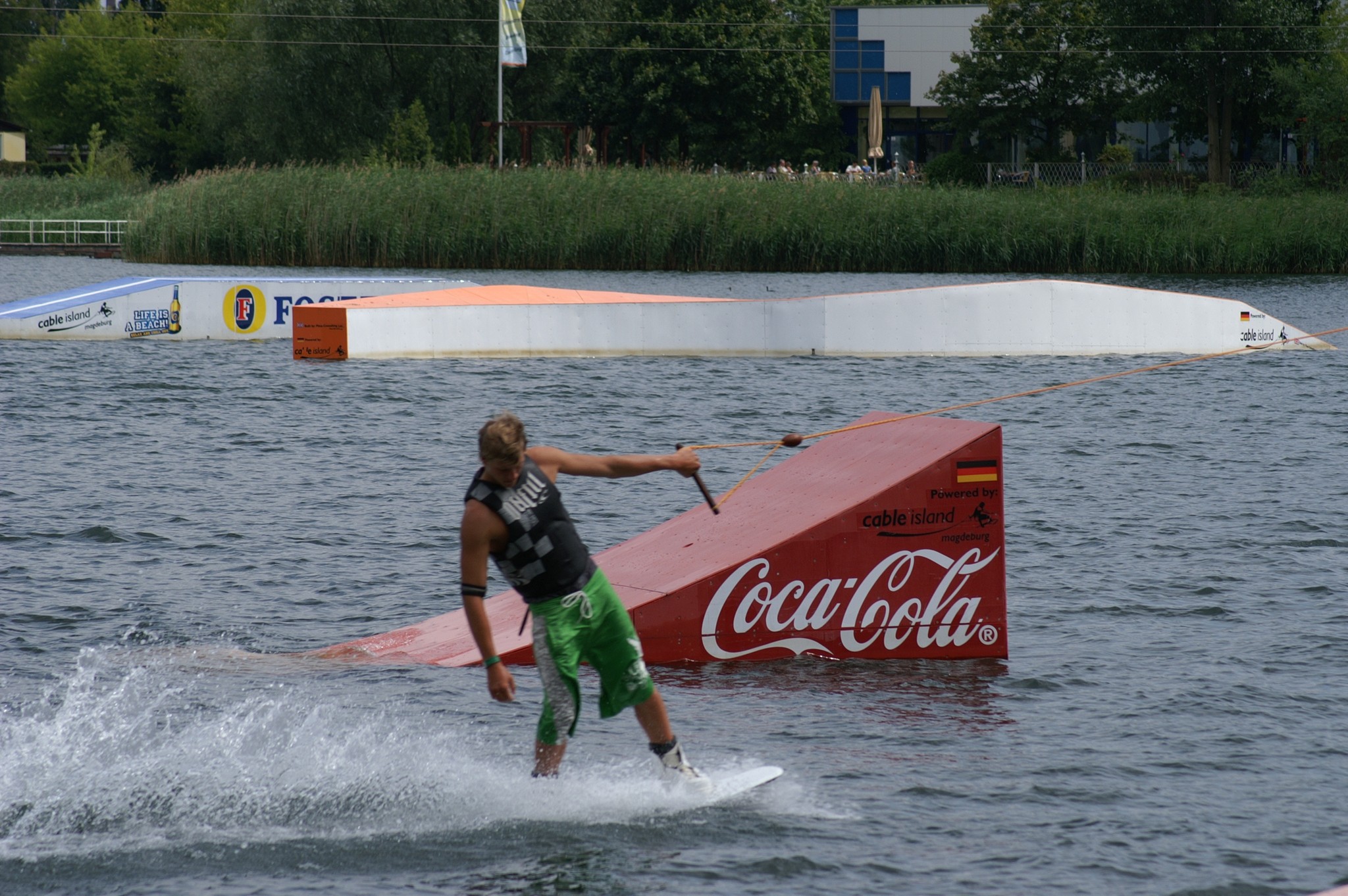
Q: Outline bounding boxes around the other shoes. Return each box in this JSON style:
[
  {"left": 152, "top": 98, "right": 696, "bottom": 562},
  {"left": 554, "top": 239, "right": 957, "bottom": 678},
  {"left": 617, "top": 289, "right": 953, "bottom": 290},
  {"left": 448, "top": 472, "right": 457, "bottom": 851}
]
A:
[{"left": 661, "top": 740, "right": 703, "bottom": 777}]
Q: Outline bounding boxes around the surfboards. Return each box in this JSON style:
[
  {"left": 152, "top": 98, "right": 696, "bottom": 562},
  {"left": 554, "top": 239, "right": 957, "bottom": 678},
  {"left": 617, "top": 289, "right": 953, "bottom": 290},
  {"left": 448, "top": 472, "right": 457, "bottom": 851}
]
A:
[{"left": 699, "top": 765, "right": 784, "bottom": 807}]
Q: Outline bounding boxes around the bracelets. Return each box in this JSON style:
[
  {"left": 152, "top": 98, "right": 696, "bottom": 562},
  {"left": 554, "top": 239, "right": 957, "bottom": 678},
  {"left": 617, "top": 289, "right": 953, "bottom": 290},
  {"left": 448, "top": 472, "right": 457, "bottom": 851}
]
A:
[{"left": 483, "top": 655, "right": 500, "bottom": 668}]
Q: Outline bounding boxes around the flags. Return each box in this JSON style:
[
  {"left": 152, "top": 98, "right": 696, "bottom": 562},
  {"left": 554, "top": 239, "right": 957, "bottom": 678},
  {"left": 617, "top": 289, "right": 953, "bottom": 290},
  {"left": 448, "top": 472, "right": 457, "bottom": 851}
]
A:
[{"left": 500, "top": 0, "right": 527, "bottom": 69}]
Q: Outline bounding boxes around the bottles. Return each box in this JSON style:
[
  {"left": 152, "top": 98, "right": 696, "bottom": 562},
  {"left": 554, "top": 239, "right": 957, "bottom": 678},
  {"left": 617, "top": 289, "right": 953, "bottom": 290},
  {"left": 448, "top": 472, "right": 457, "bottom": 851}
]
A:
[{"left": 169, "top": 285, "right": 181, "bottom": 334}]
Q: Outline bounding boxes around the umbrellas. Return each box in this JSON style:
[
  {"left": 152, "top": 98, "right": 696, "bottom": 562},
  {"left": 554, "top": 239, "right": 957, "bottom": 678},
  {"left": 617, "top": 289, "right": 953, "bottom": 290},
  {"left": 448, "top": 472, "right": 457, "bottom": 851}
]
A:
[{"left": 867, "top": 86, "right": 885, "bottom": 183}]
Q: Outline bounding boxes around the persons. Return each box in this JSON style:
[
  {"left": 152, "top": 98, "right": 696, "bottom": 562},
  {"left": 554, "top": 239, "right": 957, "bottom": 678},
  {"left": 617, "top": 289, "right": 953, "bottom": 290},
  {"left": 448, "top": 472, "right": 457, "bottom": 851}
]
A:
[
  {"left": 807, "top": 160, "right": 821, "bottom": 178},
  {"left": 460, "top": 415, "right": 708, "bottom": 780},
  {"left": 765, "top": 159, "right": 796, "bottom": 181},
  {"left": 583, "top": 144, "right": 597, "bottom": 174},
  {"left": 845, "top": 159, "right": 922, "bottom": 184}
]
